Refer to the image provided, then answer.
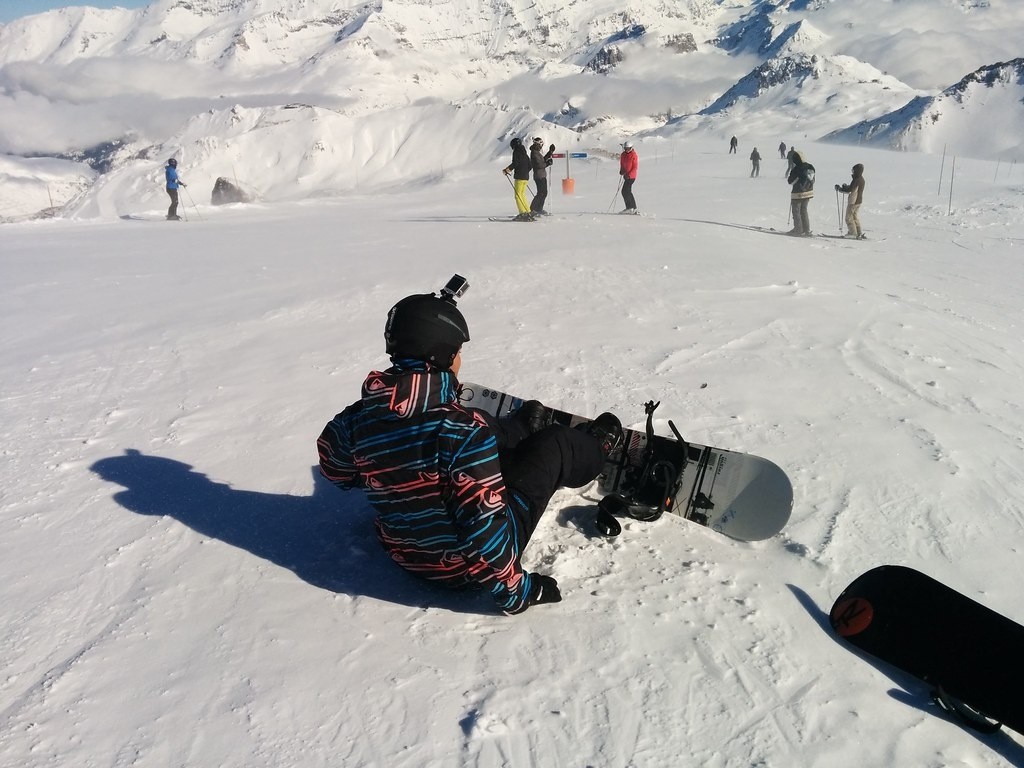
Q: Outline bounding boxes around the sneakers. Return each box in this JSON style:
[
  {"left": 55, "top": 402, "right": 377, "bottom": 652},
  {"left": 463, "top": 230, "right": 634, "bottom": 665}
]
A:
[
  {"left": 587, "top": 412, "right": 623, "bottom": 460},
  {"left": 506, "top": 400, "right": 548, "bottom": 448}
]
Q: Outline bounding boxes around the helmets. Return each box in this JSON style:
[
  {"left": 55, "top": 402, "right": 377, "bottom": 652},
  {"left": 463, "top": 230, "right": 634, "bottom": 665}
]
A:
[
  {"left": 510, "top": 138, "right": 521, "bottom": 150},
  {"left": 781, "top": 141, "right": 784, "bottom": 144},
  {"left": 168, "top": 158, "right": 177, "bottom": 167},
  {"left": 384, "top": 292, "right": 470, "bottom": 370},
  {"left": 623, "top": 141, "right": 633, "bottom": 148},
  {"left": 733, "top": 135, "right": 736, "bottom": 138},
  {"left": 533, "top": 137, "right": 544, "bottom": 149}
]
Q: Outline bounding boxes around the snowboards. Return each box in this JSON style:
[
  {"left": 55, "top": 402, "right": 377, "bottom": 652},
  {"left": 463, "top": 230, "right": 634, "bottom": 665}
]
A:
[
  {"left": 453, "top": 382, "right": 795, "bottom": 542},
  {"left": 829, "top": 564, "right": 1024, "bottom": 735}
]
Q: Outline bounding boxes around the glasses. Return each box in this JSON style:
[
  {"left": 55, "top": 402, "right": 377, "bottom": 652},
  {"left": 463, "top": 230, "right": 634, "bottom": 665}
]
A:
[{"left": 596, "top": 493, "right": 628, "bottom": 536}]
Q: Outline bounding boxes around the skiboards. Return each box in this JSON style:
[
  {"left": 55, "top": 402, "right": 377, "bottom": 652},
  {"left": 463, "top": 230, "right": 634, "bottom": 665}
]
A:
[
  {"left": 489, "top": 214, "right": 552, "bottom": 222},
  {"left": 769, "top": 227, "right": 866, "bottom": 240},
  {"left": 595, "top": 212, "right": 650, "bottom": 215}
]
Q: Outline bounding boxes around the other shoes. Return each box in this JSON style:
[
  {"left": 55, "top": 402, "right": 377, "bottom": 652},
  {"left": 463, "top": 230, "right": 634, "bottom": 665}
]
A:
[
  {"left": 512, "top": 213, "right": 534, "bottom": 221},
  {"left": 531, "top": 210, "right": 550, "bottom": 217},
  {"left": 786, "top": 231, "right": 813, "bottom": 236},
  {"left": 844, "top": 234, "right": 864, "bottom": 239},
  {"left": 167, "top": 215, "right": 181, "bottom": 220},
  {"left": 620, "top": 208, "right": 640, "bottom": 215}
]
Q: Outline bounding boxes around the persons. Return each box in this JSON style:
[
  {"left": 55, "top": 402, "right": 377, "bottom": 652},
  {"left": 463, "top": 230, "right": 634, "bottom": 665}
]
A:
[
  {"left": 505, "top": 137, "right": 555, "bottom": 222},
  {"left": 778, "top": 141, "right": 797, "bottom": 179},
  {"left": 749, "top": 147, "right": 763, "bottom": 178},
  {"left": 164, "top": 158, "right": 188, "bottom": 222},
  {"left": 835, "top": 164, "right": 866, "bottom": 238},
  {"left": 618, "top": 141, "right": 640, "bottom": 216},
  {"left": 787, "top": 151, "right": 815, "bottom": 236},
  {"left": 730, "top": 136, "right": 738, "bottom": 154},
  {"left": 316, "top": 293, "right": 623, "bottom": 617}
]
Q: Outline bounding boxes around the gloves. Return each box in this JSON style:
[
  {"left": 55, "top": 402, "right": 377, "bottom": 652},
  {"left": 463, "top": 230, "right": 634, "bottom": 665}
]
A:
[
  {"left": 834, "top": 184, "right": 840, "bottom": 191},
  {"left": 842, "top": 183, "right": 848, "bottom": 189},
  {"left": 549, "top": 144, "right": 555, "bottom": 152},
  {"left": 530, "top": 573, "right": 562, "bottom": 605},
  {"left": 503, "top": 166, "right": 512, "bottom": 175},
  {"left": 546, "top": 158, "right": 553, "bottom": 166}
]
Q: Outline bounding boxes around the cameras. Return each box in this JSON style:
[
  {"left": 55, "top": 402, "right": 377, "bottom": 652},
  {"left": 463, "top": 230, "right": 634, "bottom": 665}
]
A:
[{"left": 443, "top": 274, "right": 470, "bottom": 298}]
